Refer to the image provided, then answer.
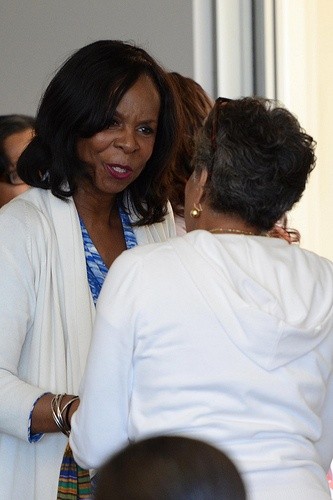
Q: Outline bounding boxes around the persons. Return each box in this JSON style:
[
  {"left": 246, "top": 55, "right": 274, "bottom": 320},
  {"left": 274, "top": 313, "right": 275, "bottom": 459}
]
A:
[
  {"left": 1, "top": 38, "right": 177, "bottom": 500},
  {"left": 1, "top": 114, "right": 37, "bottom": 202},
  {"left": 160, "top": 70, "right": 214, "bottom": 238},
  {"left": 92, "top": 435, "right": 248, "bottom": 500},
  {"left": 69, "top": 94, "right": 333, "bottom": 500}
]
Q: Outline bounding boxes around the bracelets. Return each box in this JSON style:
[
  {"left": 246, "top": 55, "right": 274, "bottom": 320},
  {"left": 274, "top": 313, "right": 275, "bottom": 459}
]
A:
[{"left": 50, "top": 393, "right": 79, "bottom": 437}]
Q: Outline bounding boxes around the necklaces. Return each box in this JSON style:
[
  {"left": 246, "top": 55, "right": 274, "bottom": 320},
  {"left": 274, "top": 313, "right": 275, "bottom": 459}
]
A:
[{"left": 210, "top": 227, "right": 253, "bottom": 235}]
